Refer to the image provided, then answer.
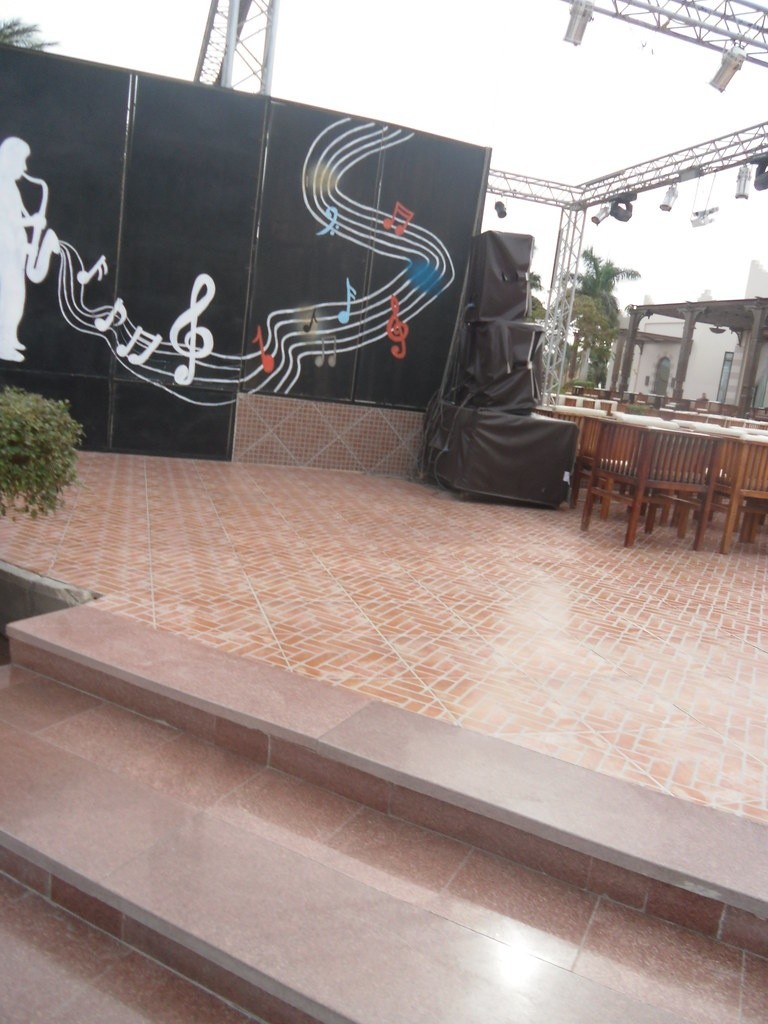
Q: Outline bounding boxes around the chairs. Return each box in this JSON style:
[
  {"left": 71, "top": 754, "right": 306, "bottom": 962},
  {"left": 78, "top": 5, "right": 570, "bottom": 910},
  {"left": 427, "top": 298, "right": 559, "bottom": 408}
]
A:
[{"left": 536, "top": 386, "right": 768, "bottom": 554}]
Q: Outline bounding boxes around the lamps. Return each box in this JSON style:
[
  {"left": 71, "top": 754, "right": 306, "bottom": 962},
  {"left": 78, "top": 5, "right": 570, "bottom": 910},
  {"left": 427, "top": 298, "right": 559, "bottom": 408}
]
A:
[
  {"left": 591, "top": 193, "right": 639, "bottom": 223},
  {"left": 494, "top": 201, "right": 507, "bottom": 218},
  {"left": 710, "top": 48, "right": 746, "bottom": 90},
  {"left": 562, "top": 0, "right": 591, "bottom": 45},
  {"left": 660, "top": 183, "right": 679, "bottom": 211},
  {"left": 734, "top": 154, "right": 768, "bottom": 200}
]
